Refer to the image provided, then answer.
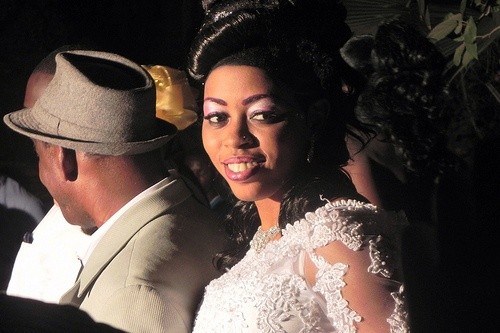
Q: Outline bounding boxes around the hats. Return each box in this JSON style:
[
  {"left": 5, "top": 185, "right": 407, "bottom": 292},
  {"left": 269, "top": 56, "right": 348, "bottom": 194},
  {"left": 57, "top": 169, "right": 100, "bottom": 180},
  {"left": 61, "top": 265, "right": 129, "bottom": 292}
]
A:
[
  {"left": 3, "top": 50, "right": 178, "bottom": 156},
  {"left": 141, "top": 65, "right": 200, "bottom": 130}
]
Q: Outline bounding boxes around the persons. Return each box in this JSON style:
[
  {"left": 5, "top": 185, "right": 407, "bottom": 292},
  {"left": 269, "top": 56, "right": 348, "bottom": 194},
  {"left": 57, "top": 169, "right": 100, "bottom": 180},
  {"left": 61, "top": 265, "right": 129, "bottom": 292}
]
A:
[
  {"left": 0, "top": 47, "right": 90, "bottom": 302},
  {"left": 0, "top": 50, "right": 231, "bottom": 333},
  {"left": 185, "top": 0, "right": 413, "bottom": 333},
  {"left": 334, "top": 105, "right": 403, "bottom": 207}
]
